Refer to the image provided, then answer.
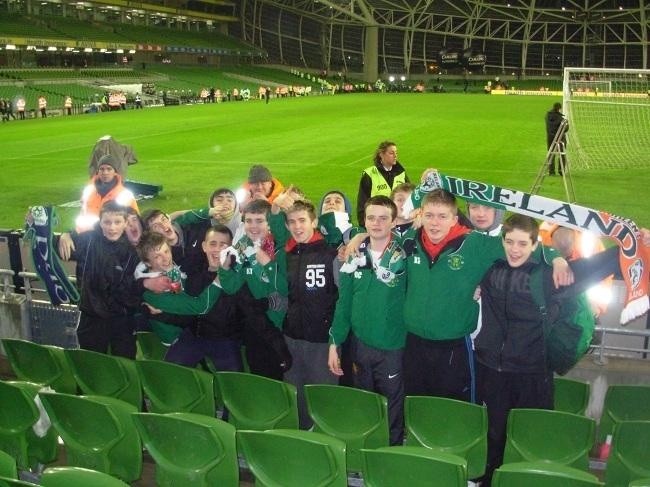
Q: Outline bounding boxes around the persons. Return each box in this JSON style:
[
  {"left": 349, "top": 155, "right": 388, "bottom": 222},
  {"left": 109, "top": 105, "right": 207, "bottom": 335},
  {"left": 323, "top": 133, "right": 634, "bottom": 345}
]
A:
[
  {"left": 540, "top": 86, "right": 598, "bottom": 93},
  {"left": 455, "top": 78, "right": 514, "bottom": 94},
  {"left": 144, "top": 84, "right": 191, "bottom": 99},
  {"left": 258, "top": 84, "right": 311, "bottom": 104},
  {"left": 0, "top": 91, "right": 72, "bottom": 121},
  {"left": 433, "top": 77, "right": 444, "bottom": 93},
  {"left": 344, "top": 189, "right": 576, "bottom": 402},
  {"left": 542, "top": 100, "right": 571, "bottom": 175},
  {"left": 56, "top": 142, "right": 612, "bottom": 431},
  {"left": 327, "top": 194, "right": 481, "bottom": 446},
  {"left": 320, "top": 79, "right": 424, "bottom": 95},
  {"left": 468, "top": 215, "right": 650, "bottom": 485},
  {"left": 200, "top": 87, "right": 250, "bottom": 103},
  {"left": 102, "top": 90, "right": 143, "bottom": 112}
]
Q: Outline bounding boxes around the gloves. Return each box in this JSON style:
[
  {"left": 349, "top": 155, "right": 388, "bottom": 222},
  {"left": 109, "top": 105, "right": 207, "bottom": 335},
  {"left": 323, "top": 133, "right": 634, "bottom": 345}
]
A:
[{"left": 270, "top": 293, "right": 288, "bottom": 312}]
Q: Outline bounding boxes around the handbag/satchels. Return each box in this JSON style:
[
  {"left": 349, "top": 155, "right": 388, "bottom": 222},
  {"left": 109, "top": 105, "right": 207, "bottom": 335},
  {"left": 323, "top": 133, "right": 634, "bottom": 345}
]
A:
[{"left": 547, "top": 296, "right": 596, "bottom": 375}]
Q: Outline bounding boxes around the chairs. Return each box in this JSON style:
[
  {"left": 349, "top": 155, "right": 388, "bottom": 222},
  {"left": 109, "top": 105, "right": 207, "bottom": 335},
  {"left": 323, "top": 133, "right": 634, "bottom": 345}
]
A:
[
  {"left": 602, "top": 418, "right": 649, "bottom": 485},
  {"left": 64, "top": 348, "right": 144, "bottom": 411},
  {"left": 132, "top": 412, "right": 239, "bottom": 486},
  {"left": 135, "top": 359, "right": 216, "bottom": 421},
  {"left": 215, "top": 371, "right": 300, "bottom": 459},
  {"left": 1, "top": 13, "right": 295, "bottom": 109},
  {"left": 1, "top": 337, "right": 78, "bottom": 396},
  {"left": 237, "top": 426, "right": 351, "bottom": 487},
  {"left": 40, "top": 466, "right": 129, "bottom": 486},
  {"left": 401, "top": 394, "right": 489, "bottom": 479},
  {"left": 295, "top": 73, "right": 649, "bottom": 96},
  {"left": 0, "top": 381, "right": 59, "bottom": 469},
  {"left": 303, "top": 384, "right": 391, "bottom": 473},
  {"left": 599, "top": 385, "right": 648, "bottom": 422},
  {"left": 490, "top": 459, "right": 603, "bottom": 486},
  {"left": 1, "top": 475, "right": 36, "bottom": 486},
  {"left": 502, "top": 408, "right": 599, "bottom": 472},
  {"left": 552, "top": 377, "right": 592, "bottom": 416},
  {"left": 1, "top": 451, "right": 18, "bottom": 478},
  {"left": 358, "top": 444, "right": 471, "bottom": 486},
  {"left": 37, "top": 390, "right": 143, "bottom": 480}
]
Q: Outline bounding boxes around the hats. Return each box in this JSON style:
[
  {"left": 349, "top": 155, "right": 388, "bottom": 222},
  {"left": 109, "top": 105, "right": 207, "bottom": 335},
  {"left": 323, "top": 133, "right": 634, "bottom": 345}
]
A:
[
  {"left": 248, "top": 164, "right": 272, "bottom": 184},
  {"left": 98, "top": 155, "right": 120, "bottom": 171},
  {"left": 320, "top": 191, "right": 351, "bottom": 217}
]
